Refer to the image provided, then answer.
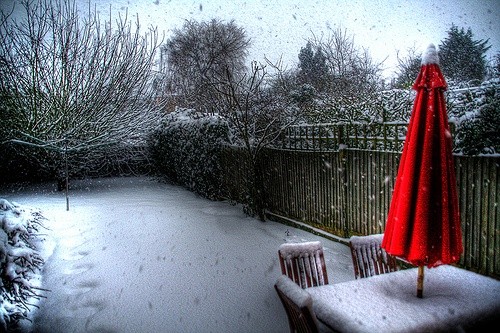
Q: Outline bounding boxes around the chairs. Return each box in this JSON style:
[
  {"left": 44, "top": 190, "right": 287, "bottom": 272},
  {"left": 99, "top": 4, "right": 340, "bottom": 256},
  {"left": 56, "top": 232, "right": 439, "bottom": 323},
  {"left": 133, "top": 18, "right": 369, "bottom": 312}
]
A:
[
  {"left": 274, "top": 283, "right": 318, "bottom": 332},
  {"left": 350, "top": 234, "right": 398, "bottom": 281},
  {"left": 278, "top": 245, "right": 329, "bottom": 289}
]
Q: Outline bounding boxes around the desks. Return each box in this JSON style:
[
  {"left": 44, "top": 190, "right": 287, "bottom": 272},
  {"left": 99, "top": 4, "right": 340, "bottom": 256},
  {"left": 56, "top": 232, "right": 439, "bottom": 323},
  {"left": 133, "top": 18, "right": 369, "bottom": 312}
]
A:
[{"left": 305, "top": 263, "right": 497, "bottom": 333}]
